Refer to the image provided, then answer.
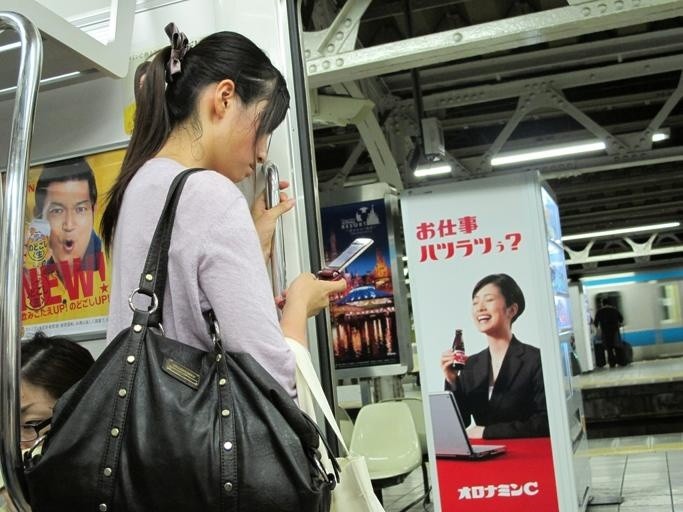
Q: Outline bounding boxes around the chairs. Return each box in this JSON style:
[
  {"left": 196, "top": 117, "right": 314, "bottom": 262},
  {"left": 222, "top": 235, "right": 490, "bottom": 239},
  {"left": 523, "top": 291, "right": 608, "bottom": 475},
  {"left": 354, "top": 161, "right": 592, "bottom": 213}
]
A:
[{"left": 327, "top": 395, "right": 434, "bottom": 512}]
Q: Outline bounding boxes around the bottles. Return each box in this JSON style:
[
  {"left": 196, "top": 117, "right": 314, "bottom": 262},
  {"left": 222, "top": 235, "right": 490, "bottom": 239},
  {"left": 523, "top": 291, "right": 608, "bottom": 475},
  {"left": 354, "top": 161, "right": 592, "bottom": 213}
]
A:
[{"left": 448, "top": 330, "right": 465, "bottom": 372}]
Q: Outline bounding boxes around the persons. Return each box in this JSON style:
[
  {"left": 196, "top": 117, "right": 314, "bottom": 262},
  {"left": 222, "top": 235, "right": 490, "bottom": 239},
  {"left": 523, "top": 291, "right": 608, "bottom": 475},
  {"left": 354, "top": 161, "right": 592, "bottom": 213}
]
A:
[
  {"left": 23, "top": 154, "right": 100, "bottom": 275},
  {"left": 0, "top": 334, "right": 104, "bottom": 511},
  {"left": 98, "top": 30, "right": 352, "bottom": 512},
  {"left": 438, "top": 272, "right": 549, "bottom": 441},
  {"left": 592, "top": 297, "right": 624, "bottom": 369}
]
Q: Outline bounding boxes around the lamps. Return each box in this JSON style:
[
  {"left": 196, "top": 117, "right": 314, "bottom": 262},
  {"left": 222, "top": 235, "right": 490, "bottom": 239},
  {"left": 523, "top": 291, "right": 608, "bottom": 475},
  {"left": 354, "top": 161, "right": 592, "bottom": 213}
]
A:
[{"left": 405, "top": 123, "right": 673, "bottom": 183}]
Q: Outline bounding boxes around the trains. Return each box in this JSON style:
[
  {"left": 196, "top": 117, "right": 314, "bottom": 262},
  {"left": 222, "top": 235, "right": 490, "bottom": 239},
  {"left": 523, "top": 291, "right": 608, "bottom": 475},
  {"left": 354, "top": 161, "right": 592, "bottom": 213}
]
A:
[{"left": 577, "top": 267, "right": 683, "bottom": 362}]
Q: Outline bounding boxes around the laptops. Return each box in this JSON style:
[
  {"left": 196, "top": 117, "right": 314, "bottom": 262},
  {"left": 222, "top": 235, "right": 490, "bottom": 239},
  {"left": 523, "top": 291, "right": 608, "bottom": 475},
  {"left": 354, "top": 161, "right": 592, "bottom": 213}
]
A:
[{"left": 428, "top": 391, "right": 506, "bottom": 461}]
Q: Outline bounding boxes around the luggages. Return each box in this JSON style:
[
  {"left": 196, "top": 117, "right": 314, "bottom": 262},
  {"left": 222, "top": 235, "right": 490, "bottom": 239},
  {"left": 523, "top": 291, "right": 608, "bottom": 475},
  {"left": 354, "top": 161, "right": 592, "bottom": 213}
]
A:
[{"left": 613, "top": 324, "right": 632, "bottom": 366}]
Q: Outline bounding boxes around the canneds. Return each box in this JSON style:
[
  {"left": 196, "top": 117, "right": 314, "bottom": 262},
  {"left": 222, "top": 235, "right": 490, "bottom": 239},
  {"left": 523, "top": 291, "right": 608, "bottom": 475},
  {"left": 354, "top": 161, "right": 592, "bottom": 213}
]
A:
[{"left": 23, "top": 219, "right": 51, "bottom": 270}]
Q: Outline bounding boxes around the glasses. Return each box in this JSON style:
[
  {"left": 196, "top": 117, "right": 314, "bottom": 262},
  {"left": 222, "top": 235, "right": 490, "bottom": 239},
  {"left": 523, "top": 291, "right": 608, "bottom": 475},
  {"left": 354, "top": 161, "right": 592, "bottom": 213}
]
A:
[{"left": 18, "top": 417, "right": 53, "bottom": 443}]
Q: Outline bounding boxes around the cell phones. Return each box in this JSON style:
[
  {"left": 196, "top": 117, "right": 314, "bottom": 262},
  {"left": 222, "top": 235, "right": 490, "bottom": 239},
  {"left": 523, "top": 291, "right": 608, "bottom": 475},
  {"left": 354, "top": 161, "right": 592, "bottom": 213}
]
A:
[{"left": 275, "top": 237, "right": 374, "bottom": 309}]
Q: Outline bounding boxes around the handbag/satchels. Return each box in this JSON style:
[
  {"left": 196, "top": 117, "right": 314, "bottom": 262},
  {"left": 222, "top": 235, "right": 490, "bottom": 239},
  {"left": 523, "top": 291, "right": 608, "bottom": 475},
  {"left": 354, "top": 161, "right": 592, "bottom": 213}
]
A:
[{"left": 22, "top": 324, "right": 343, "bottom": 512}]
